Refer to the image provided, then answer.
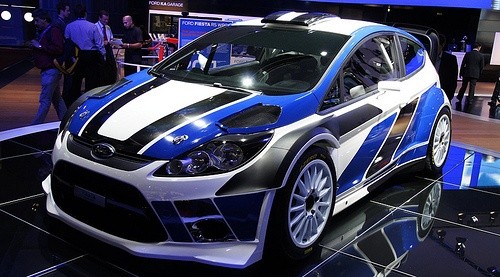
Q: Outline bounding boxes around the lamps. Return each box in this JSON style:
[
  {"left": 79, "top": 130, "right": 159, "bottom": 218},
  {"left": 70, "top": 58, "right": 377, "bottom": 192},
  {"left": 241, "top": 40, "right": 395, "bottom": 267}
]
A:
[
  {"left": 436, "top": 229, "right": 446, "bottom": 241},
  {"left": 488, "top": 266, "right": 499, "bottom": 276},
  {"left": 455, "top": 237, "right": 467, "bottom": 254},
  {"left": 457, "top": 212, "right": 464, "bottom": 223},
  {"left": 489, "top": 211, "right": 496, "bottom": 224}
]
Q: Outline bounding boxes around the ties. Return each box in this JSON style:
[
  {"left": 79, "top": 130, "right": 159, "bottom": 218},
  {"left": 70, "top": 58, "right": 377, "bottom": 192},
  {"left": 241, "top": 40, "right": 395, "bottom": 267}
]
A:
[{"left": 102, "top": 25, "right": 108, "bottom": 42}]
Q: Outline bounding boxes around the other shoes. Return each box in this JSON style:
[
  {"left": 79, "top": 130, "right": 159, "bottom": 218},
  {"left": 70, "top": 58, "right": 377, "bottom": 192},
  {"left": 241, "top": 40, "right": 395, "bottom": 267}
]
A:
[
  {"left": 469, "top": 102, "right": 473, "bottom": 104},
  {"left": 457, "top": 95, "right": 462, "bottom": 100}
]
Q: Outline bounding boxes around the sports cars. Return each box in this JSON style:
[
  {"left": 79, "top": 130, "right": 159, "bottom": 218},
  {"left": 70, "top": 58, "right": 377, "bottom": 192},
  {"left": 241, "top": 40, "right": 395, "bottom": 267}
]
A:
[
  {"left": 264, "top": 163, "right": 445, "bottom": 277},
  {"left": 42, "top": 11, "right": 454, "bottom": 268}
]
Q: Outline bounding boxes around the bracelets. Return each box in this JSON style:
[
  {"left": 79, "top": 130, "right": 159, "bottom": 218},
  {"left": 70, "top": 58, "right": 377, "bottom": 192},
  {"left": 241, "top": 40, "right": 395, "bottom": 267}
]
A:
[
  {"left": 127, "top": 44, "right": 130, "bottom": 48},
  {"left": 38, "top": 44, "right": 42, "bottom": 48}
]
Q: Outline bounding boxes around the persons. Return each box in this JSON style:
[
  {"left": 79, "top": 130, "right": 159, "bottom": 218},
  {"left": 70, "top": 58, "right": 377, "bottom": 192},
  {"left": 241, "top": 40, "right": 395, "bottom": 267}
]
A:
[
  {"left": 119, "top": 16, "right": 143, "bottom": 77},
  {"left": 456, "top": 42, "right": 484, "bottom": 104},
  {"left": 95, "top": 11, "right": 118, "bottom": 85},
  {"left": 488, "top": 70, "right": 500, "bottom": 105},
  {"left": 51, "top": 2, "right": 70, "bottom": 31},
  {"left": 431, "top": 34, "right": 458, "bottom": 105},
  {"left": 29, "top": 8, "right": 68, "bottom": 125},
  {"left": 447, "top": 38, "right": 458, "bottom": 52},
  {"left": 65, "top": 4, "right": 106, "bottom": 98}
]
